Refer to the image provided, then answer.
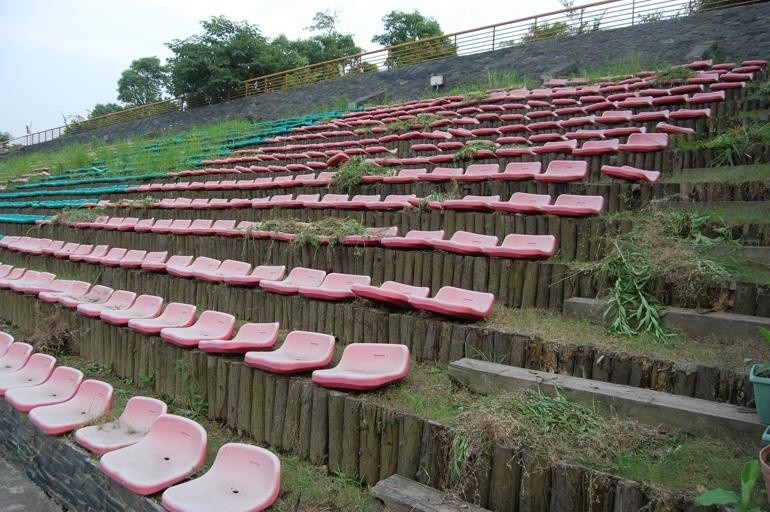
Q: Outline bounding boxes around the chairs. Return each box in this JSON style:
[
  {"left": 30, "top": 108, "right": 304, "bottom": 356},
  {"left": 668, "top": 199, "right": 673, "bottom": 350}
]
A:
[
  {"left": 0, "top": 332, "right": 282, "bottom": 512},
  {"left": 0, "top": 192, "right": 604, "bottom": 390},
  {"left": 0, "top": 59, "right": 767, "bottom": 196}
]
